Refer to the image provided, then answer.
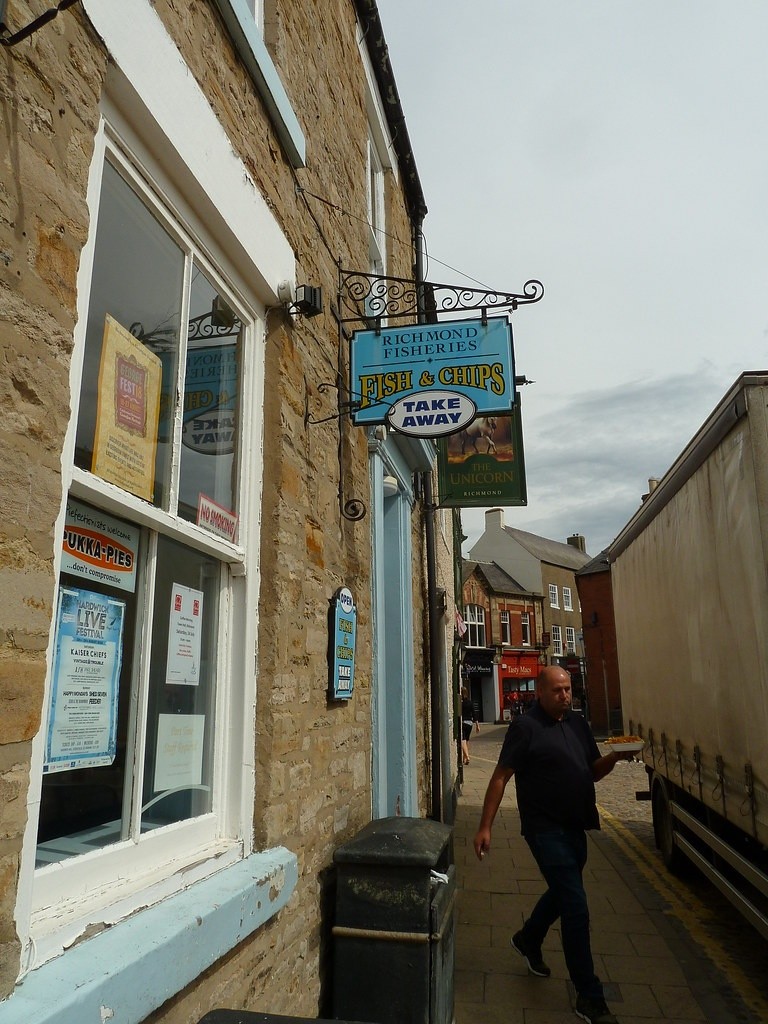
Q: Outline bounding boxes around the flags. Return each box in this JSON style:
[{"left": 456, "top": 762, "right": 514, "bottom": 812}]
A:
[{"left": 453, "top": 606, "right": 467, "bottom": 638}]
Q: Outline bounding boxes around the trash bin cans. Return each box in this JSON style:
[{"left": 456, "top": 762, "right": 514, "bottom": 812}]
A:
[
  {"left": 572, "top": 709, "right": 583, "bottom": 719},
  {"left": 332, "top": 816, "right": 458, "bottom": 1024}
]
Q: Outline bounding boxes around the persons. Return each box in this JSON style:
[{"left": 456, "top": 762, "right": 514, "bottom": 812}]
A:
[
  {"left": 474, "top": 666, "right": 639, "bottom": 1024},
  {"left": 460, "top": 688, "right": 480, "bottom": 766}
]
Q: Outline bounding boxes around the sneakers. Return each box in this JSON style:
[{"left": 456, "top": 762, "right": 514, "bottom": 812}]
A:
[
  {"left": 510, "top": 933, "right": 550, "bottom": 977},
  {"left": 575, "top": 1008, "right": 618, "bottom": 1023}
]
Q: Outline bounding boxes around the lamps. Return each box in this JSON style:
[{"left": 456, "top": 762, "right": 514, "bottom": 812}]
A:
[
  {"left": 211, "top": 296, "right": 236, "bottom": 327},
  {"left": 287, "top": 283, "right": 324, "bottom": 319}
]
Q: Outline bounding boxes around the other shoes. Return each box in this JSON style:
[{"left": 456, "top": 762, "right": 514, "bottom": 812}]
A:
[{"left": 465, "top": 760, "right": 470, "bottom": 765}]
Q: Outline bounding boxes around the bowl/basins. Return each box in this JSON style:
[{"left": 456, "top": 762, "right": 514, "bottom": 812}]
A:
[{"left": 609, "top": 739, "right": 645, "bottom": 751}]
